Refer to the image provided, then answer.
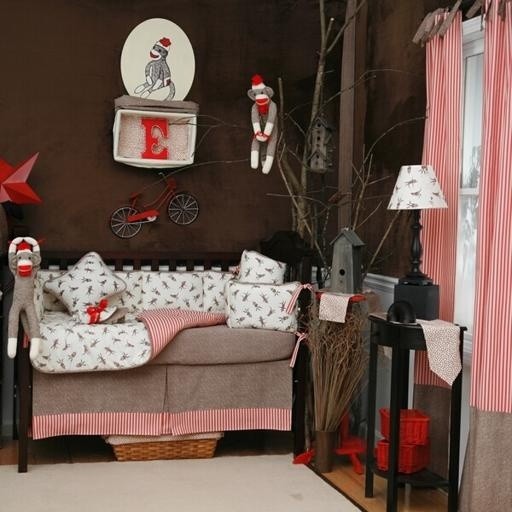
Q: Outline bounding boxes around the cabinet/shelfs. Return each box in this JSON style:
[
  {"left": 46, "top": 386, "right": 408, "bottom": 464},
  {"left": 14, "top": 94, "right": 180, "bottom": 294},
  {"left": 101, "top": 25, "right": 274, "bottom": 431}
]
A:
[{"left": 366, "top": 312, "right": 468, "bottom": 512}]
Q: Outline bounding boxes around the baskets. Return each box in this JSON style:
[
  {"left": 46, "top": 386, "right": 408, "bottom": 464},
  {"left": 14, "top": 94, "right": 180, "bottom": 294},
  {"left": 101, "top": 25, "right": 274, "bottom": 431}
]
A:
[
  {"left": 375, "top": 405, "right": 435, "bottom": 476},
  {"left": 103, "top": 431, "right": 225, "bottom": 462}
]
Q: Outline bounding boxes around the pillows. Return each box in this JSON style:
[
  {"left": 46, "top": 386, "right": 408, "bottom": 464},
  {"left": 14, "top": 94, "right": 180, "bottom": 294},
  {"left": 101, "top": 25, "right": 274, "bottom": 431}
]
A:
[
  {"left": 47, "top": 250, "right": 124, "bottom": 315},
  {"left": 219, "top": 249, "right": 304, "bottom": 331}
]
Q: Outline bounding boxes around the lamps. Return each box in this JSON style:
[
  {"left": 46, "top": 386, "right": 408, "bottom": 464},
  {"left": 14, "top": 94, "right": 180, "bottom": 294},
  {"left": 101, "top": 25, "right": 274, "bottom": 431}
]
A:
[{"left": 381, "top": 158, "right": 450, "bottom": 322}]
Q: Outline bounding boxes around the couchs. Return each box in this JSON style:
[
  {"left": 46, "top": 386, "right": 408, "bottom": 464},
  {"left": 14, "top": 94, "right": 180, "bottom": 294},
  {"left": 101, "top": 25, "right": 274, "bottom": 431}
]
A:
[{"left": 10, "top": 242, "right": 342, "bottom": 471}]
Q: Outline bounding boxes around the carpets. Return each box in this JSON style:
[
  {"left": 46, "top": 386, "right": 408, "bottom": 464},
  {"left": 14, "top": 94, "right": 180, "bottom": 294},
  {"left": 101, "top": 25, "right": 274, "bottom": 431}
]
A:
[{"left": 0, "top": 453, "right": 362, "bottom": 512}]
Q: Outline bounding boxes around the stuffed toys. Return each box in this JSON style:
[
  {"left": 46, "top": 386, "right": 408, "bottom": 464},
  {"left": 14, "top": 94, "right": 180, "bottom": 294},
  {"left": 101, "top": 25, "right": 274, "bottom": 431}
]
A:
[
  {"left": 7, "top": 236, "right": 42, "bottom": 361},
  {"left": 247, "top": 74, "right": 279, "bottom": 175}
]
